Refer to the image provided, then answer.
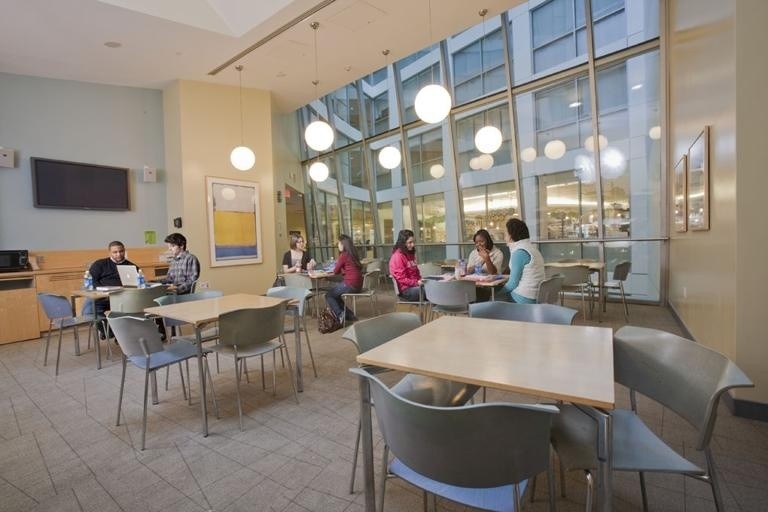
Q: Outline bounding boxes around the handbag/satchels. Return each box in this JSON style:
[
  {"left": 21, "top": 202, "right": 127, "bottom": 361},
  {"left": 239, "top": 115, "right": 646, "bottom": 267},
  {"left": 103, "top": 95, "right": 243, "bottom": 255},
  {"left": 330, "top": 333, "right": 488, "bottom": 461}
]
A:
[{"left": 318, "top": 310, "right": 340, "bottom": 334}]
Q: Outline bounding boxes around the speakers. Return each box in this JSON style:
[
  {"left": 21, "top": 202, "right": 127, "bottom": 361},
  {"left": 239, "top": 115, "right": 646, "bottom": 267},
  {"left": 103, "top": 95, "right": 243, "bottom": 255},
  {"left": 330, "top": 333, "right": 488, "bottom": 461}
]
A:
[
  {"left": 144, "top": 167, "right": 156, "bottom": 183},
  {"left": 0, "top": 149, "right": 15, "bottom": 168}
]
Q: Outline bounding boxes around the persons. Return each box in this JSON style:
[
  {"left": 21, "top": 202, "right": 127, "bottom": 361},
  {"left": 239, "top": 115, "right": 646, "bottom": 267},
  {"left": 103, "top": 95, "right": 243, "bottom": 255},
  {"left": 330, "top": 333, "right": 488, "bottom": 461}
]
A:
[
  {"left": 148, "top": 232, "right": 200, "bottom": 341},
  {"left": 493, "top": 218, "right": 546, "bottom": 303},
  {"left": 388, "top": 229, "right": 427, "bottom": 303},
  {"left": 324, "top": 234, "right": 363, "bottom": 326},
  {"left": 281, "top": 234, "right": 317, "bottom": 274},
  {"left": 80, "top": 240, "right": 146, "bottom": 345},
  {"left": 466, "top": 228, "right": 504, "bottom": 276}
]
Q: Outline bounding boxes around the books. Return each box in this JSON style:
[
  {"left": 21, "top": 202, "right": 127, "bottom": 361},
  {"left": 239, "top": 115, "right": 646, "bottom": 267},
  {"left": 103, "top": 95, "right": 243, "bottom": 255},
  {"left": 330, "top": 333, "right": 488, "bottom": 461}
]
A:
[{"left": 96, "top": 285, "right": 125, "bottom": 293}]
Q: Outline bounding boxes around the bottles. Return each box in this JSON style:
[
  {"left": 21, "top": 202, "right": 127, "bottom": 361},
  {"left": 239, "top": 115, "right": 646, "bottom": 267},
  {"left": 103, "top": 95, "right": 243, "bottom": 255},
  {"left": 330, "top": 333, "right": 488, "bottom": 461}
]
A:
[
  {"left": 136, "top": 269, "right": 145, "bottom": 289},
  {"left": 475, "top": 260, "right": 482, "bottom": 275},
  {"left": 455, "top": 259, "right": 466, "bottom": 279},
  {"left": 84, "top": 271, "right": 93, "bottom": 290},
  {"left": 329, "top": 257, "right": 335, "bottom": 271},
  {"left": 296, "top": 261, "right": 302, "bottom": 273}
]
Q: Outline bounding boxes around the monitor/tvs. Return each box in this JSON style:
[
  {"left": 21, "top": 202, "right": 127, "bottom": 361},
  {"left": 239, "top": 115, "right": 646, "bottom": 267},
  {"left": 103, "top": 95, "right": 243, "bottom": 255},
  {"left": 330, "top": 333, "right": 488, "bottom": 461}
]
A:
[{"left": 31, "top": 157, "right": 130, "bottom": 211}]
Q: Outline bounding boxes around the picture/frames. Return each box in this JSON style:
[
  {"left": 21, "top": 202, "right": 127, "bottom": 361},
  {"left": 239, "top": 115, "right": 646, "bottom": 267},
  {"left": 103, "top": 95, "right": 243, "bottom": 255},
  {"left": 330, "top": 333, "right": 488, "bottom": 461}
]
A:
[
  {"left": 205, "top": 177, "right": 262, "bottom": 268},
  {"left": 672, "top": 125, "right": 710, "bottom": 233}
]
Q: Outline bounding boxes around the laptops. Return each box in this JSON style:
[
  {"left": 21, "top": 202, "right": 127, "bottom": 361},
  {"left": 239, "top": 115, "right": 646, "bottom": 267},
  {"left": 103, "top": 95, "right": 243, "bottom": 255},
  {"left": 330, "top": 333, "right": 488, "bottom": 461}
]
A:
[{"left": 116, "top": 263, "right": 162, "bottom": 290}]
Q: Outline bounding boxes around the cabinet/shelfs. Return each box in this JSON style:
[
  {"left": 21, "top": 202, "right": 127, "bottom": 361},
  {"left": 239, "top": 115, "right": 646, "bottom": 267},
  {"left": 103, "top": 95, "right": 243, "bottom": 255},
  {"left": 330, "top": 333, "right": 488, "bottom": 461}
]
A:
[{"left": 0, "top": 262, "right": 174, "bottom": 344}]
